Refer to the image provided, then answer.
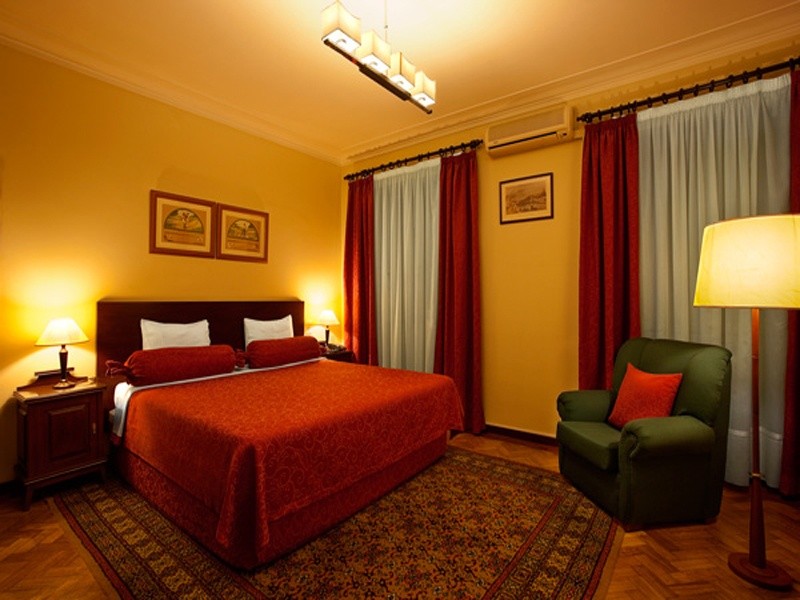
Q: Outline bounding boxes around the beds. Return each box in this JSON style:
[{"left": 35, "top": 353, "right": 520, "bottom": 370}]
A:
[{"left": 95, "top": 301, "right": 464, "bottom": 571}]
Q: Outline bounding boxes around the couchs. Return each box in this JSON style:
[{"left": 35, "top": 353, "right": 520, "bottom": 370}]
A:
[{"left": 556, "top": 336, "right": 733, "bottom": 533}]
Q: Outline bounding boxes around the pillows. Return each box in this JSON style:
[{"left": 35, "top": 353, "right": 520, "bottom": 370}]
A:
[
  {"left": 243, "top": 314, "right": 294, "bottom": 349},
  {"left": 140, "top": 319, "right": 211, "bottom": 349},
  {"left": 238, "top": 335, "right": 327, "bottom": 371},
  {"left": 105, "top": 344, "right": 238, "bottom": 386},
  {"left": 608, "top": 362, "right": 683, "bottom": 426}
]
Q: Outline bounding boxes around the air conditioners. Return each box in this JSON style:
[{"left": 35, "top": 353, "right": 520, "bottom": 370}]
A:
[{"left": 485, "top": 105, "right": 575, "bottom": 158}]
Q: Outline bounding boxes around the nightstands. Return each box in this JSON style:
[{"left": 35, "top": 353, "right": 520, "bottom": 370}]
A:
[
  {"left": 13, "top": 368, "right": 108, "bottom": 513},
  {"left": 327, "top": 349, "right": 354, "bottom": 362}
]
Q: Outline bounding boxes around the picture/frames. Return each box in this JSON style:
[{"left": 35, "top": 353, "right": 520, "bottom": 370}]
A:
[
  {"left": 149, "top": 190, "right": 216, "bottom": 258},
  {"left": 498, "top": 172, "right": 554, "bottom": 225},
  {"left": 217, "top": 203, "right": 269, "bottom": 263}
]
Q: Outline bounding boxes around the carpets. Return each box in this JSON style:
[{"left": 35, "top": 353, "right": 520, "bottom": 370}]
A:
[{"left": 47, "top": 446, "right": 624, "bottom": 600}]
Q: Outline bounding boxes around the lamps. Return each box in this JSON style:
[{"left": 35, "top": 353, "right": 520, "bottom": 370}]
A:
[
  {"left": 35, "top": 317, "right": 90, "bottom": 389},
  {"left": 692, "top": 213, "right": 800, "bottom": 588},
  {"left": 320, "top": 0, "right": 436, "bottom": 114},
  {"left": 317, "top": 310, "right": 340, "bottom": 345}
]
